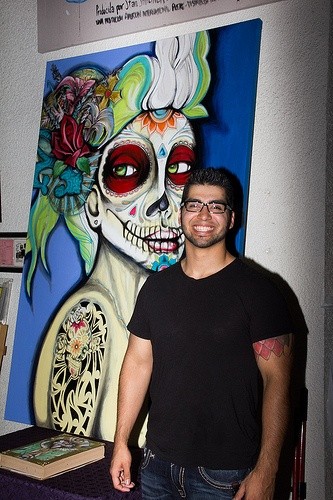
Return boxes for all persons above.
[109,167,308,500]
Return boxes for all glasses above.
[181,199,232,214]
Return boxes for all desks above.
[0,419,143,500]
